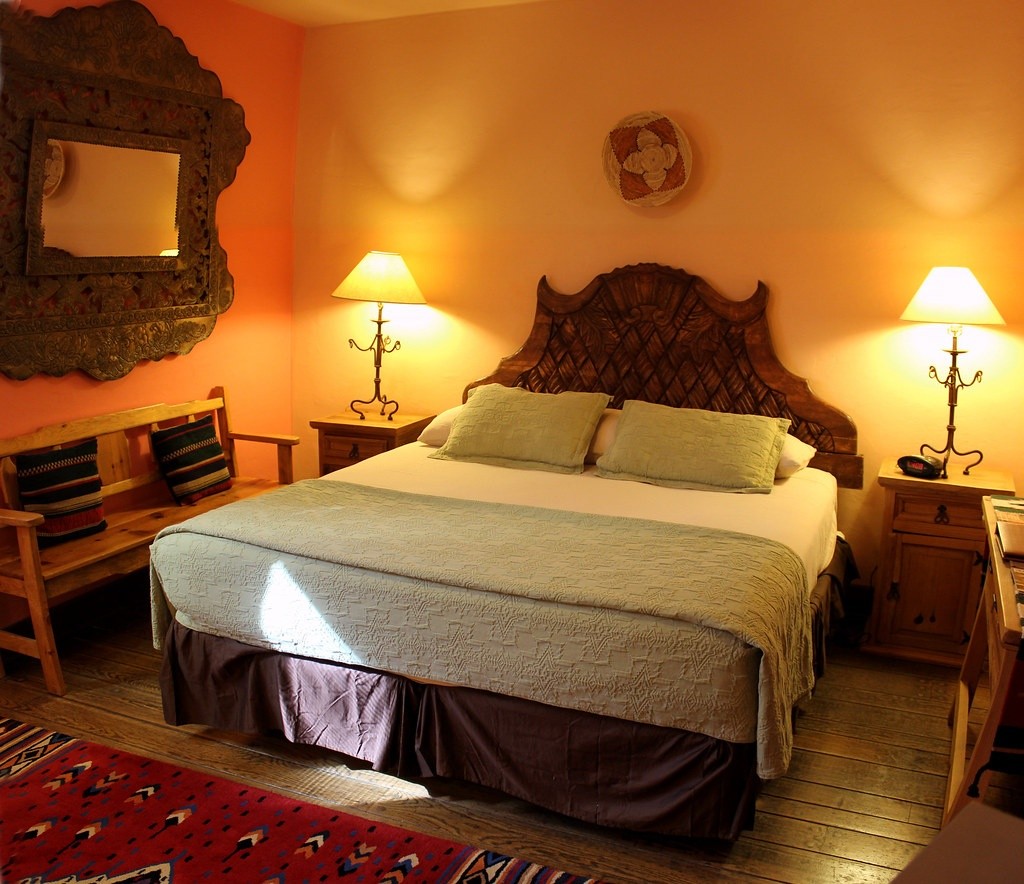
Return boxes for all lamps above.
[900,266,1006,478]
[330,250,428,422]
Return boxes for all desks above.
[939,494,1024,827]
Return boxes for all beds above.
[142,261,866,868]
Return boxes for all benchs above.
[0,386,302,698]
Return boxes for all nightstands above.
[859,457,1017,668]
[309,410,437,479]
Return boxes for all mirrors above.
[0,0,253,384]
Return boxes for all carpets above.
[0,715,603,884]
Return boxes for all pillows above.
[417,403,818,482]
[8,439,109,551]
[427,383,615,477]
[150,414,233,509]
[592,400,793,494]
